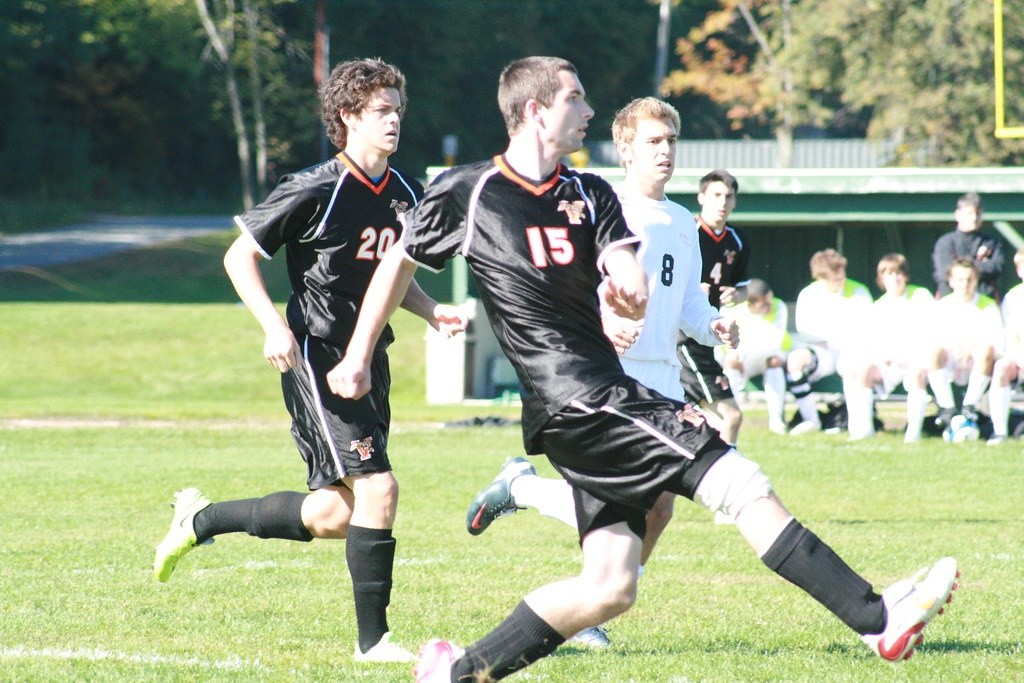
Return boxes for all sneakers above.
[935,407,959,428]
[862,556,961,663]
[153,488,216,584]
[465,454,537,535]
[961,405,979,422]
[567,627,612,646]
[354,631,417,661]
[414,637,466,683]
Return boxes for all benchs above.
[746,371,1024,402]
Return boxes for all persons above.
[716,248,1024,446]
[327,57,962,683]
[154,58,469,664]
[676,170,745,524]
[932,193,1007,305]
[466,96,739,652]
